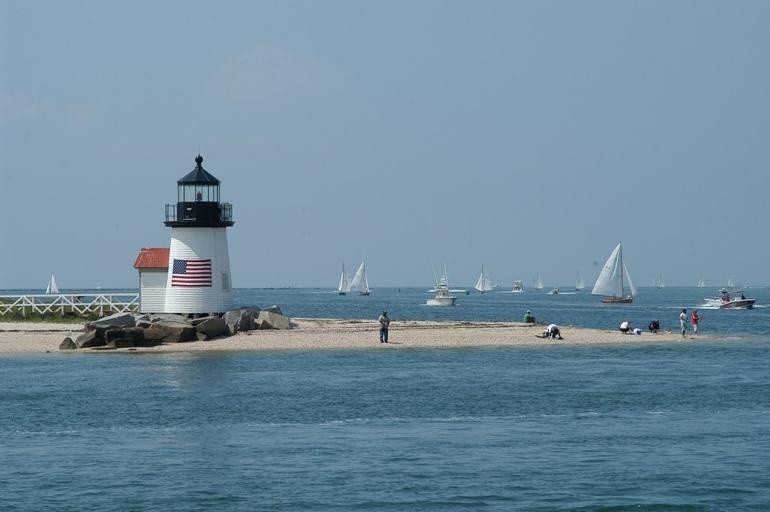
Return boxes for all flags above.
[171,258,213,289]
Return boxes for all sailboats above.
[46,273,60,294]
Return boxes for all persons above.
[523,308,536,323]
[378,309,390,343]
[544,324,564,340]
[619,320,630,334]
[690,309,700,338]
[679,308,689,339]
[648,319,660,334]
